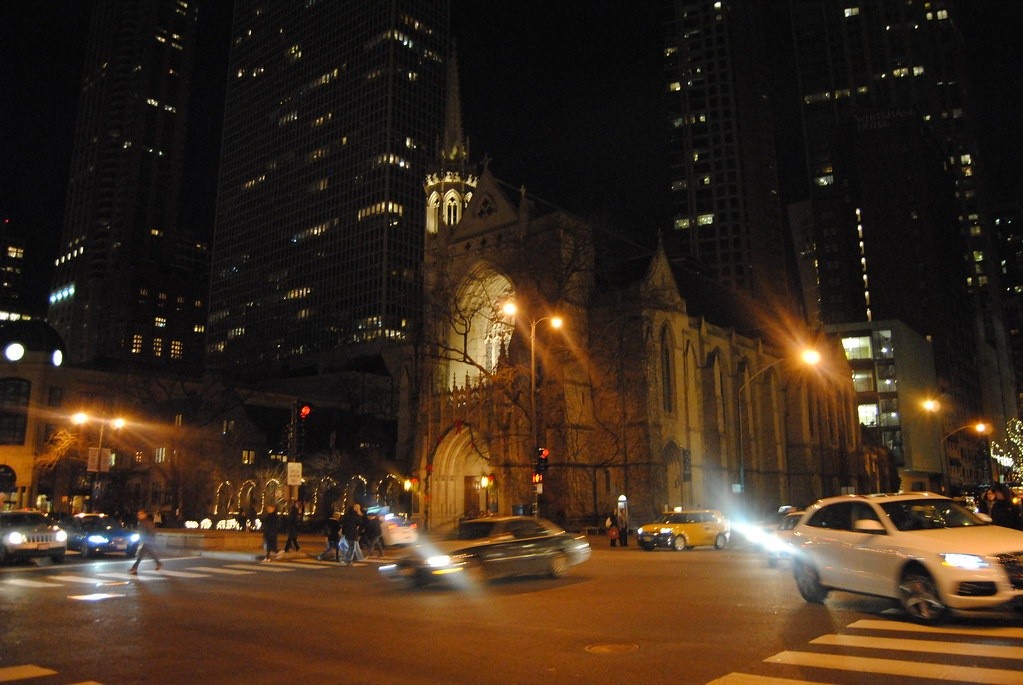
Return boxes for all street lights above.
[877,398,933,492]
[503,300,562,519]
[940,422,984,495]
[737,347,820,507]
[72,411,125,514]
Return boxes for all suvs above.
[790,490,1023,625]
[636,509,730,551]
[0,511,67,567]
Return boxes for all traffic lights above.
[532,472,543,483]
[297,402,311,437]
[536,448,548,473]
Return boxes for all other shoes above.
[261,558,270,562]
[155,562,162,570]
[274,550,283,557]
[127,566,136,574]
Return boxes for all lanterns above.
[487,475,494,481]
[302,406,310,414]
[533,472,543,483]
[424,493,431,501]
[410,476,418,484]
[543,448,549,456]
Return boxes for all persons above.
[618,508,628,546]
[991,484,1023,531]
[284,506,301,553]
[356,509,371,549]
[980,489,999,515]
[128,508,164,573]
[342,503,366,564]
[361,513,385,558]
[317,512,342,562]
[607,524,619,547]
[610,508,618,526]
[260,504,282,562]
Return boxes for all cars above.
[394,516,591,590]
[749,511,806,568]
[53,513,140,559]
[338,515,417,549]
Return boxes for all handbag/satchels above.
[337,536,348,553]
[609,528,619,540]
